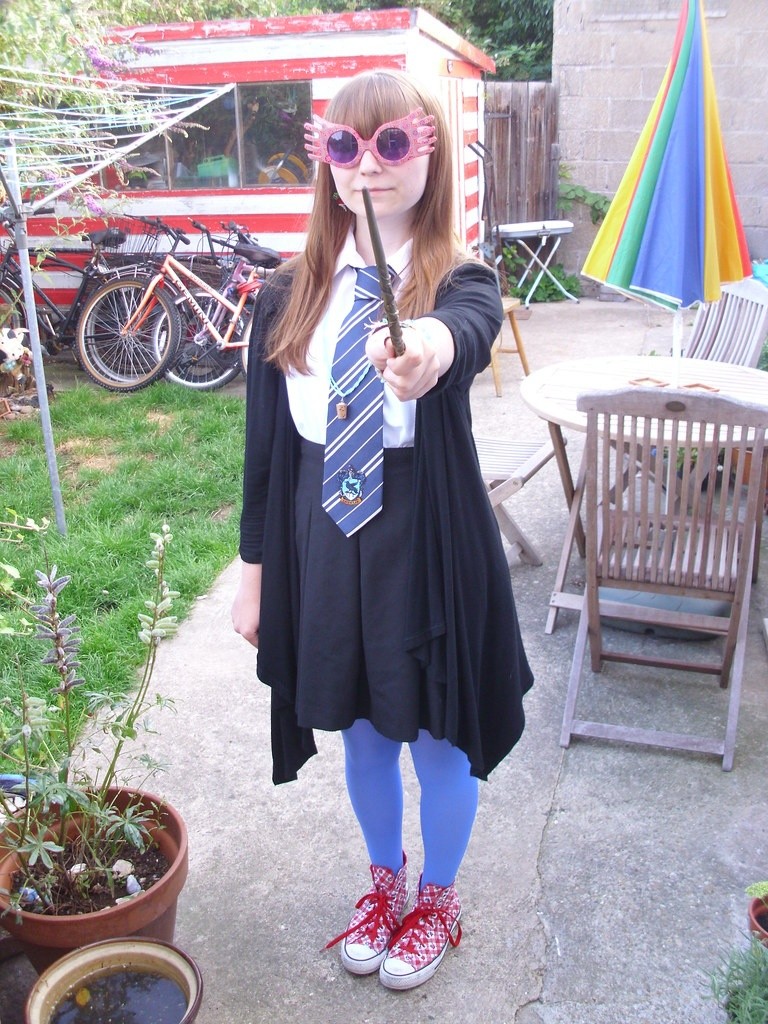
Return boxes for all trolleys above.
[466,139,533,322]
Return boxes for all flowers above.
[0,507,189,926]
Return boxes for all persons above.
[232,74,534,992]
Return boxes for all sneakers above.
[380,873,462,990]
[319,850,410,974]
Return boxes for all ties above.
[322,265,399,538]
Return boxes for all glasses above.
[303,105,437,170]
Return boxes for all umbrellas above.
[580,0,755,385]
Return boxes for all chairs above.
[487,297,531,397]
[597,278,768,519]
[559,386,768,773]
[473,434,567,566]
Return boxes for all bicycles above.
[0,206,289,393]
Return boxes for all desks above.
[491,221,580,309]
[520,355,768,634]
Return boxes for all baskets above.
[105,211,161,263]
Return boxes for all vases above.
[0,786,190,977]
[25,937,203,1024]
[731,448,768,488]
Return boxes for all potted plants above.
[663,446,726,491]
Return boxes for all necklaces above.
[329,363,371,420]
